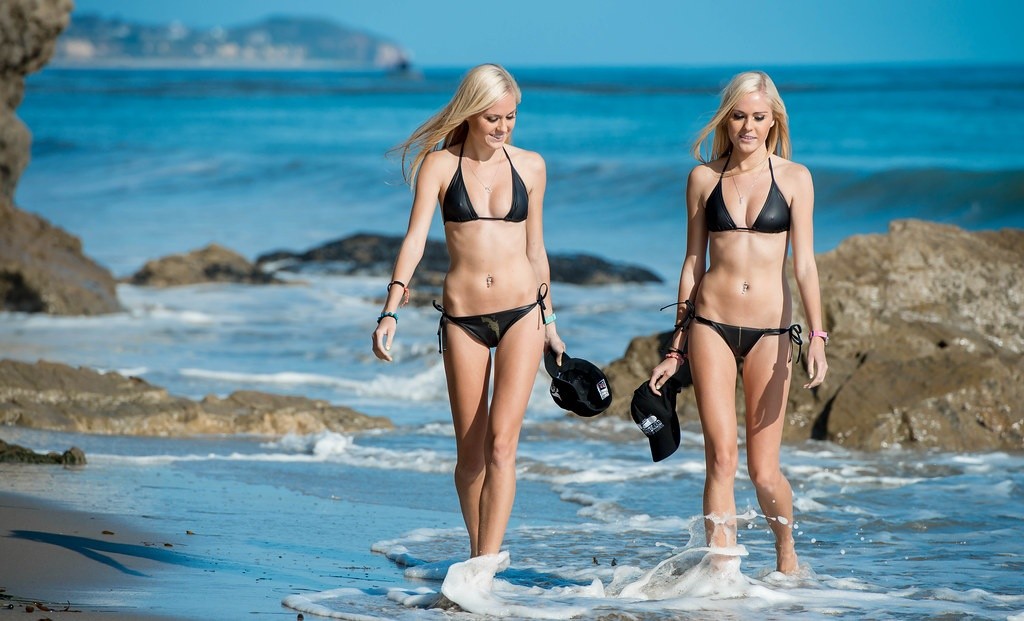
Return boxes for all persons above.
[372,64,566,559]
[649,71,828,587]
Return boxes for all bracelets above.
[808,330,828,346]
[665,345,686,365]
[544,313,556,324]
[387,280,409,308]
[377,312,398,323]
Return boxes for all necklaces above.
[461,147,504,193]
[730,158,768,203]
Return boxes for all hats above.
[631,375,683,462]
[544,349,612,417]
